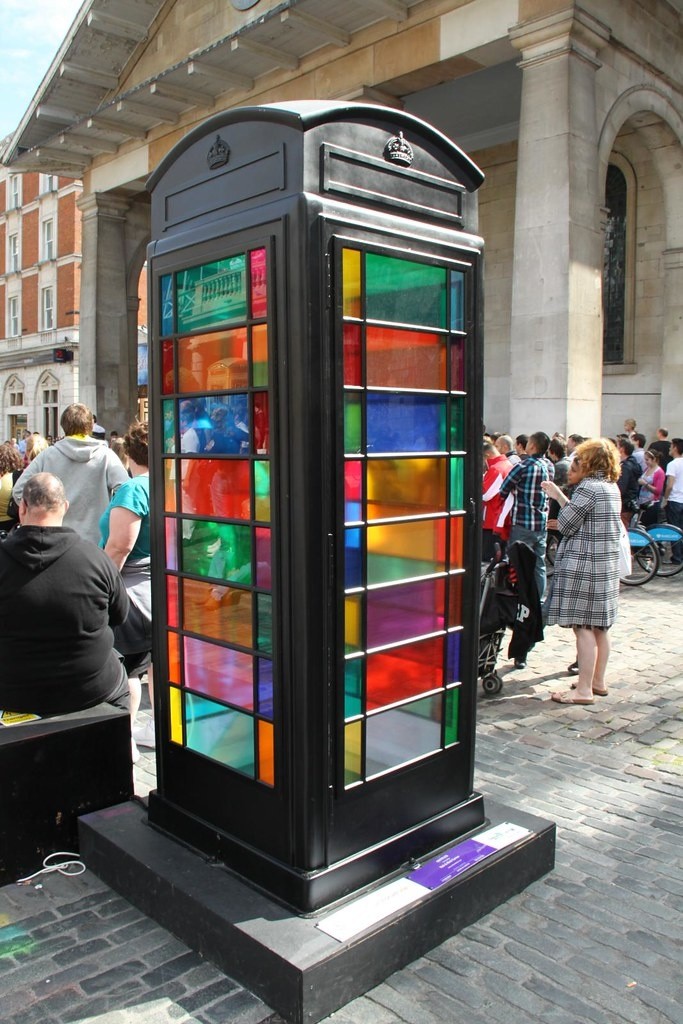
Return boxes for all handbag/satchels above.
[7,470,24,519]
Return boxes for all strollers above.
[481,541,522,693]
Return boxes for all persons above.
[164,397,275,611]
[482,417,683,705]
[0,403,153,764]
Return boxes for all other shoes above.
[567,663,579,676]
[514,658,527,669]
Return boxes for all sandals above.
[551,691,596,705]
[570,682,609,697]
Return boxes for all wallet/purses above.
[637,498,659,510]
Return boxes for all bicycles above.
[546,527,661,585]
[625,499,683,577]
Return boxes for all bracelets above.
[645,483,649,487]
